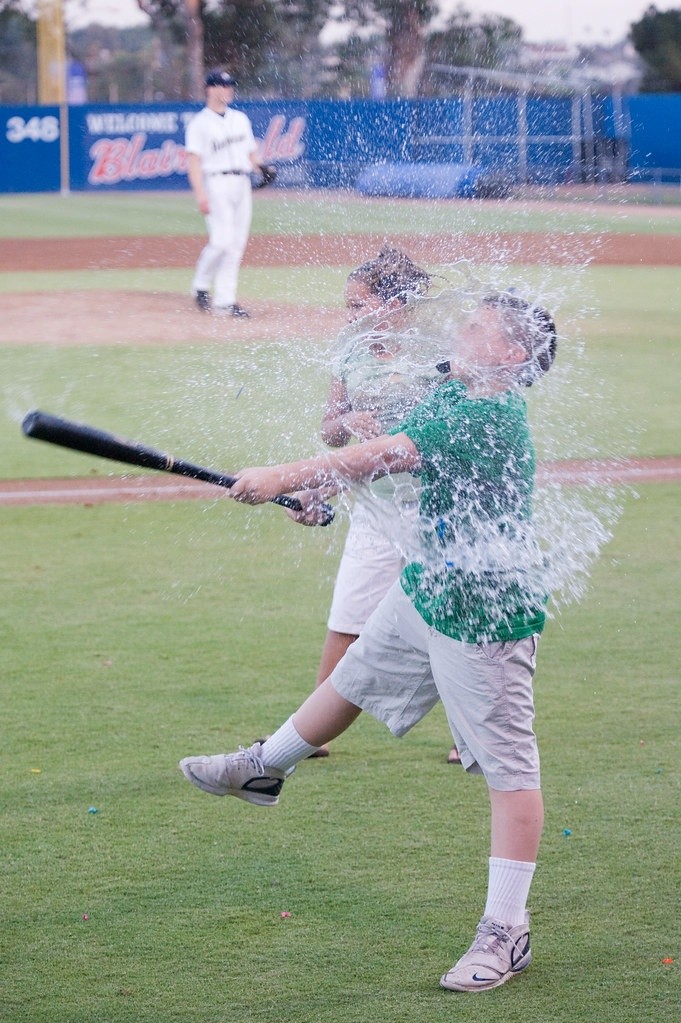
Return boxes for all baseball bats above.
[20,409,335,529]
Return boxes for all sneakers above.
[440,910,533,992]
[179,742,296,806]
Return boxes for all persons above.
[181,293,558,995]
[183,72,278,318]
[245,249,468,765]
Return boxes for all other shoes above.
[254,737,330,757]
[216,304,251,319]
[195,290,212,315]
[448,745,459,765]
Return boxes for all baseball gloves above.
[253,163,278,190]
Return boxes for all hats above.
[205,70,236,86]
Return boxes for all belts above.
[221,169,246,175]
